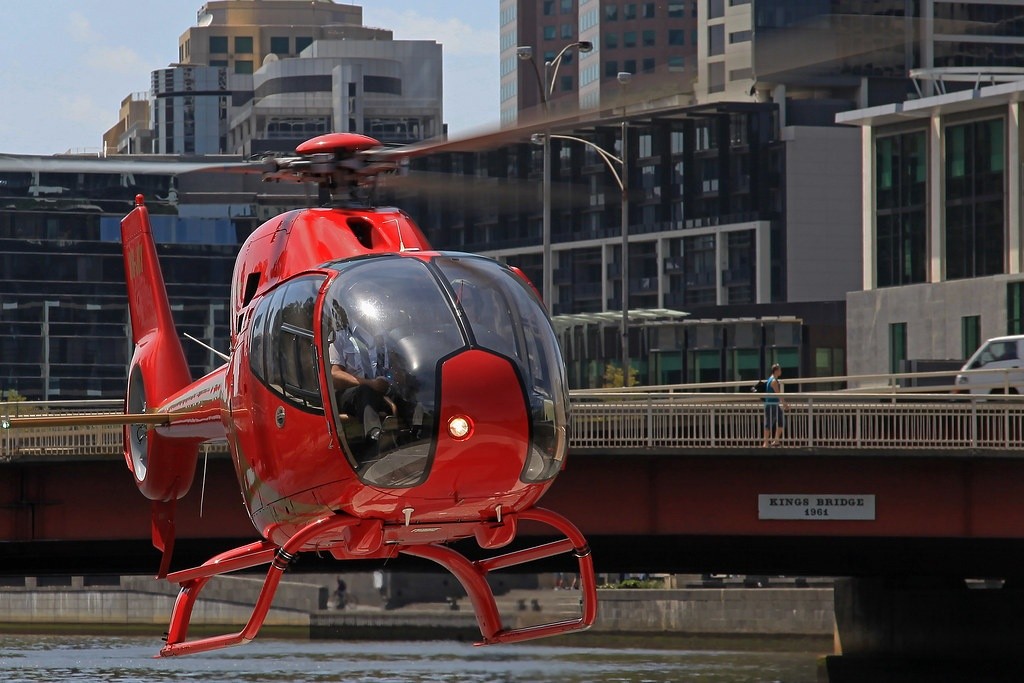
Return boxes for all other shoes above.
[367,429,381,440]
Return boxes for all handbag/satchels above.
[754,382,769,402]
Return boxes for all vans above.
[950,333,1024,405]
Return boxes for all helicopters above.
[0,1,1001,660]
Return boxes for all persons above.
[988,342,1017,361]
[763,364,789,448]
[327,298,423,450]
[421,280,533,439]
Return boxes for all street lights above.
[529,71,634,387]
[514,39,594,324]
